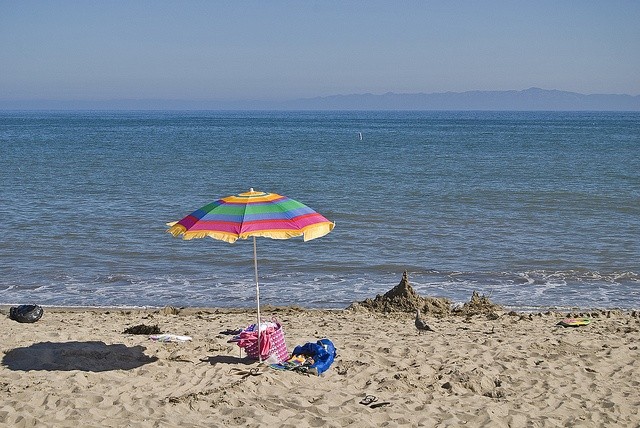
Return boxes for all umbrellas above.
[165,187,336,364]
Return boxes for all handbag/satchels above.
[228,317,292,366]
